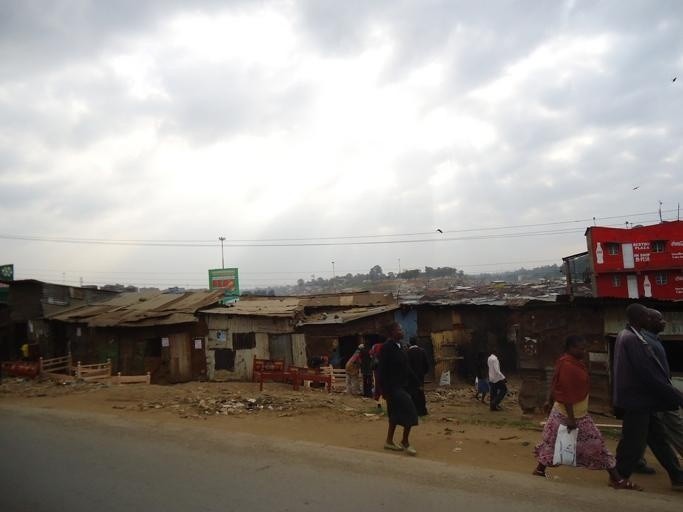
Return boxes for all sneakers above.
[607,459,656,490]
[531,468,546,477]
[383,441,417,456]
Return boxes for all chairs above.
[319,366,350,390]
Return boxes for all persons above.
[327,323,433,454]
[532,335,643,492]
[475,357,489,406]
[634,309,682,474]
[487,346,507,410]
[610,303,683,490]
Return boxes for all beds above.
[250,354,300,392]
[40,349,110,382]
[74,354,153,384]
[285,359,330,393]
